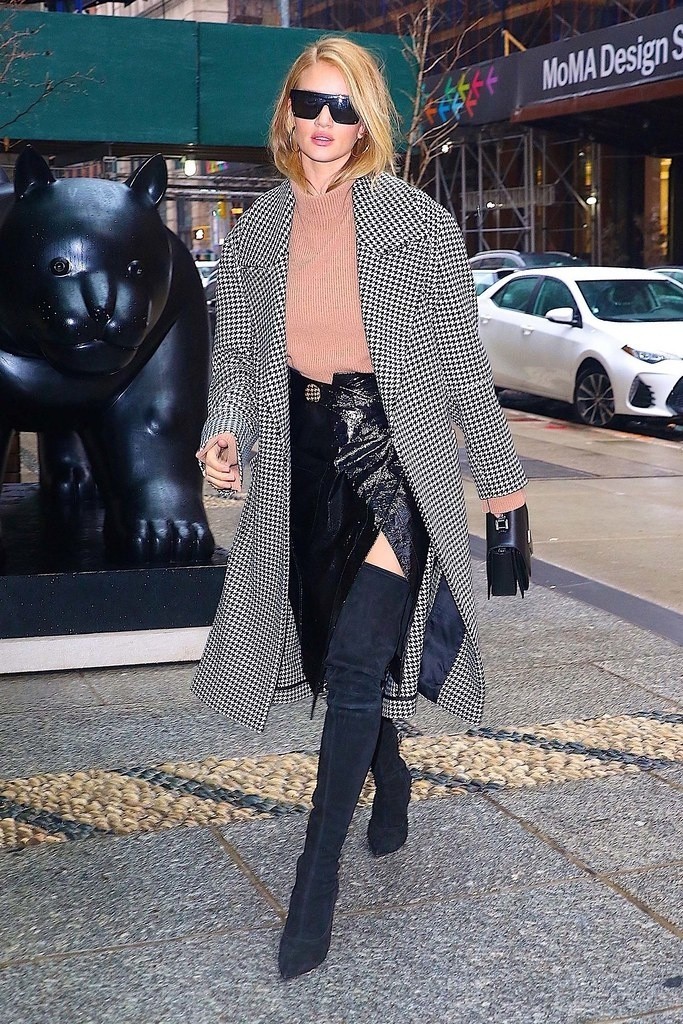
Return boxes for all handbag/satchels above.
[486,503,533,600]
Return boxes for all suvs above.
[469,249,598,310]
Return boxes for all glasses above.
[288,89,363,125]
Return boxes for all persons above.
[196,34,532,985]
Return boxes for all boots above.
[277,561,419,980]
[369,716,411,857]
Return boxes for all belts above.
[289,373,364,416]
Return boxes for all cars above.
[477,266,683,435]
